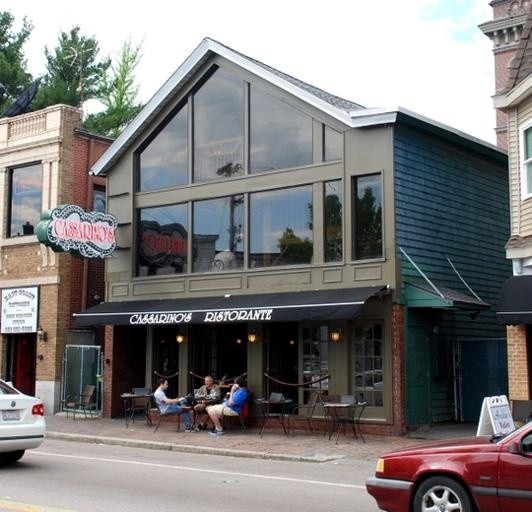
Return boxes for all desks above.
[255,399,292,436]
[186,399,218,433]
[324,402,369,444]
[121,393,156,425]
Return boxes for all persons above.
[205,376,248,436]
[194,376,221,432]
[153,378,200,432]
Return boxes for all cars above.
[1,377,47,467]
[312,369,384,393]
[366,416,532,511]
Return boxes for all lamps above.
[37,325,47,343]
[176,334,184,344]
[329,327,343,344]
[247,329,257,343]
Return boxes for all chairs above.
[318,395,348,437]
[208,259,224,271]
[125,396,150,428]
[287,393,319,437]
[221,398,247,433]
[153,398,180,433]
[67,385,95,419]
